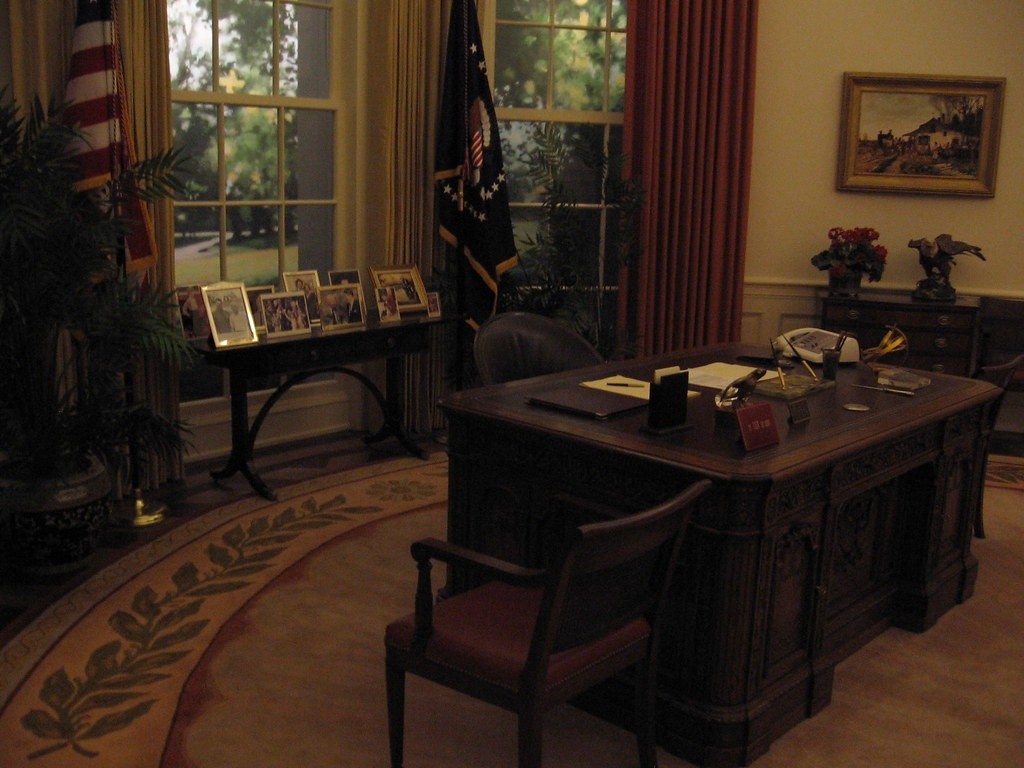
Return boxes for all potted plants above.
[0,82,204,577]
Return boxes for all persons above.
[381,289,396,317]
[182,279,362,337]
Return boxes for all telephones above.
[776,328,859,364]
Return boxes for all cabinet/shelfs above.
[814,290,982,377]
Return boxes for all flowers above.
[810,227,887,283]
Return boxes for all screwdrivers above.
[852,384,914,396]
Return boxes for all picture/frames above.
[367,263,428,314]
[316,283,365,331]
[199,282,259,348]
[835,72,1007,198]
[282,270,322,323]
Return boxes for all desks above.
[437,340,1005,768]
[183,310,461,502]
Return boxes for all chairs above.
[384,480,713,768]
[969,353,1024,539]
[469,311,605,388]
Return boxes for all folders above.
[525,386,649,420]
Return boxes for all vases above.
[828,269,863,296]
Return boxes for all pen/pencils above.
[835,330,849,351]
[781,333,816,378]
[770,338,786,390]
[607,382,645,388]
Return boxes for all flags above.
[60,0,160,288]
[436,0,516,334]
